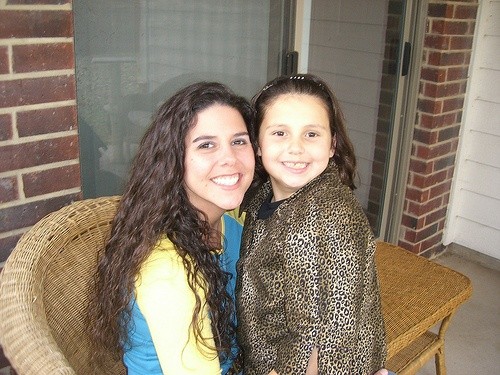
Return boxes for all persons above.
[237,73,385,375]
[80,82,388,375]
